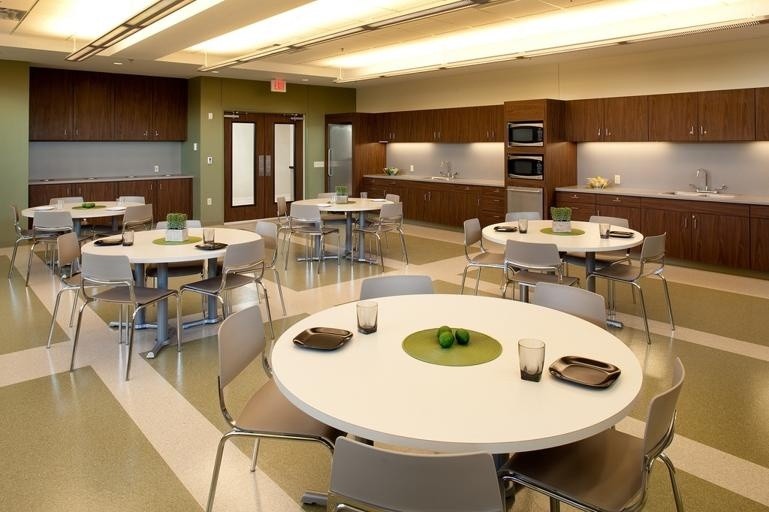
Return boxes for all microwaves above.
[508,154,544,180]
[509,121,543,147]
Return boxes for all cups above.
[361,192,367,200]
[202,229,214,243]
[56,200,63,210]
[115,198,124,207]
[329,195,335,204]
[354,301,378,335]
[121,230,133,246]
[518,338,545,382]
[599,224,610,240]
[517,218,528,234]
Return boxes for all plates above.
[292,327,353,351]
[371,199,386,202]
[314,203,330,207]
[193,243,227,250]
[548,355,620,389]
[93,238,121,246]
[29,205,55,211]
[608,230,634,238]
[493,225,516,232]
[105,207,126,211]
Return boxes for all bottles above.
[439,160,448,177]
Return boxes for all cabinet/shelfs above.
[361,178,505,231]
[455,105,504,143]
[28,177,192,218]
[560,96,649,141]
[756,88,769,141]
[649,90,756,144]
[688,200,751,272]
[751,205,769,275]
[365,113,410,142]
[411,108,455,143]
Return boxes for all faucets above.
[696,168,708,186]
[440,160,451,177]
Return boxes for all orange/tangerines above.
[82,203,95,208]
[437,326,470,348]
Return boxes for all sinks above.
[698,193,737,198]
[657,190,701,196]
[424,177,464,182]
